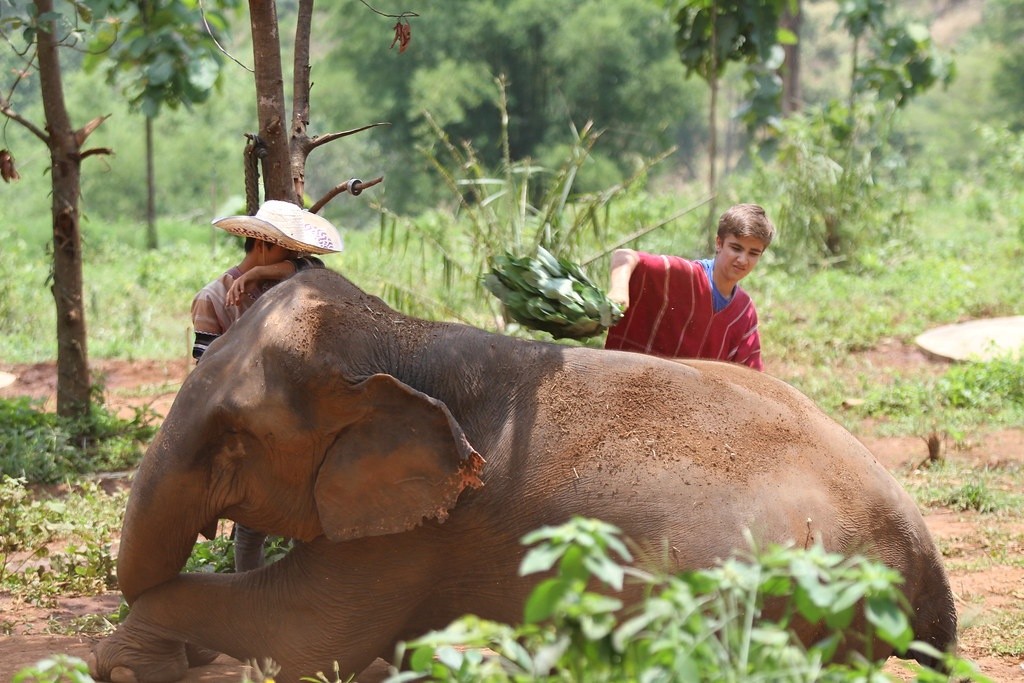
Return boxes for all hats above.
[211,200,343,255]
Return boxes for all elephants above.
[89,268,958,682]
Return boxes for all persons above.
[605,203,773,373]
[191,199,343,574]
